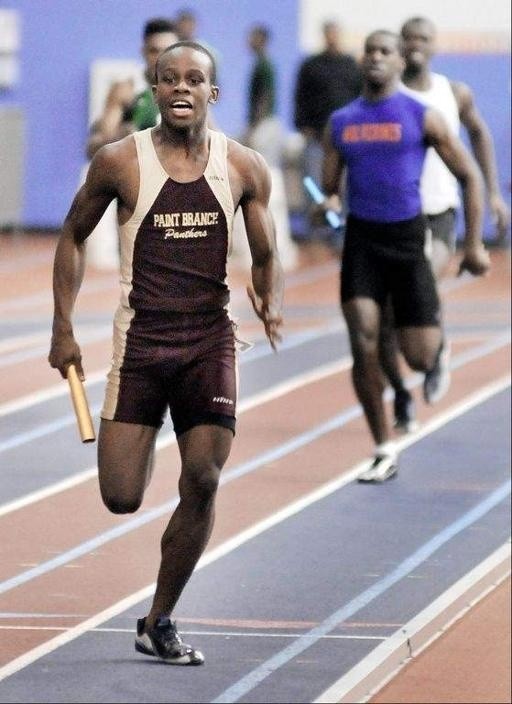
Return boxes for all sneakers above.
[391,391,418,434]
[355,442,399,482]
[423,335,452,405]
[133,614,206,666]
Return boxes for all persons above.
[48,39,284,665]
[176,7,362,273]
[311,30,491,482]
[394,19,510,277]
[86,17,220,160]
